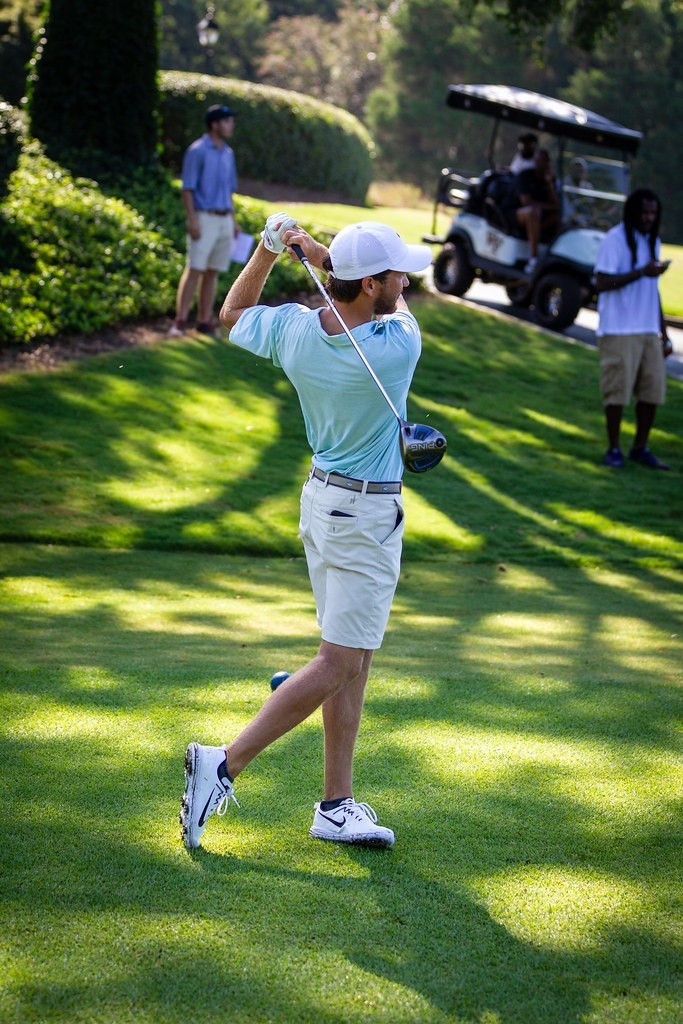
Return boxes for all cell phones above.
[662,260,672,266]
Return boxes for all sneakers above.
[178,742,240,848]
[309,798,394,848]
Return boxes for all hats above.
[327,221,433,281]
[518,133,537,145]
[205,104,241,126]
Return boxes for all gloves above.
[261,211,297,255]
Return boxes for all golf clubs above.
[276,214,455,481]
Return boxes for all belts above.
[206,209,231,216]
[310,465,401,494]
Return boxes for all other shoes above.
[525,259,539,275]
[628,445,669,470]
[196,323,220,338]
[604,448,624,469]
[168,326,185,339]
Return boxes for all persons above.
[594,189,674,470]
[168,103,241,338]
[177,212,432,851]
[472,132,560,273]
